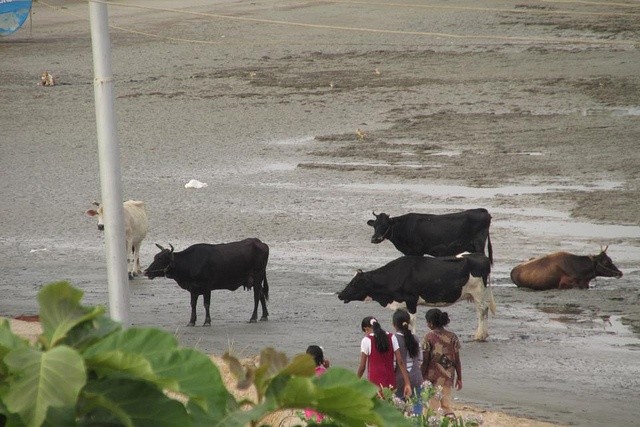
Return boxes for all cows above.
[143,238,269,327]
[367,208,494,264]
[340,251,496,341]
[510,244,623,289]
[87,200,148,280]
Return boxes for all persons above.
[421,309,464,419]
[393,309,425,416]
[304,345,330,424]
[356,316,412,400]
